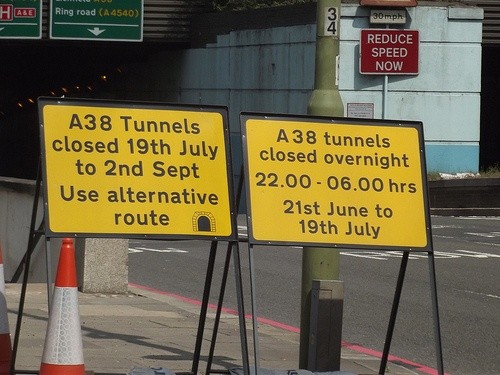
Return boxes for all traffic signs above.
[0,0,143,43]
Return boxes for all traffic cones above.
[39,237,86,375]
[0,244,16,374]
